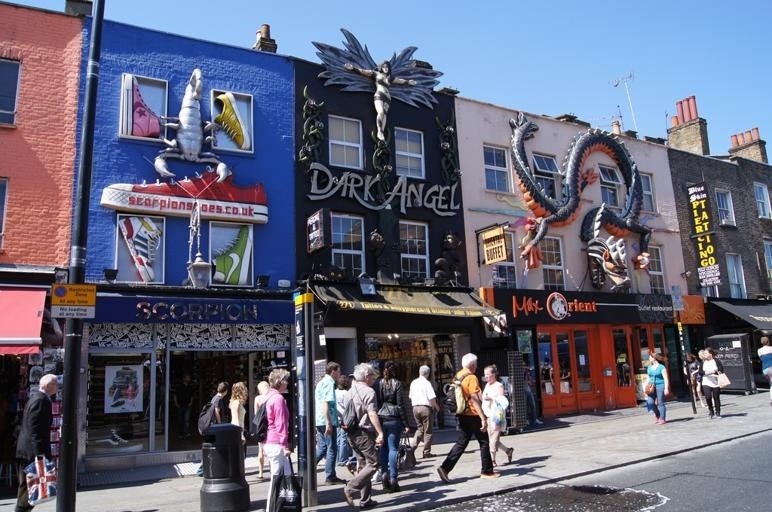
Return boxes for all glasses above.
[50,381,59,386]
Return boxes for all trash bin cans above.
[200,424,250,512]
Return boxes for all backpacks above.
[249,392,276,443]
[340,386,365,434]
[443,372,473,416]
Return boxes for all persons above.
[14,373,59,512]
[698,348,724,420]
[683,352,705,409]
[229,382,249,448]
[408,364,440,459]
[698,350,704,362]
[171,374,193,440]
[481,365,514,467]
[343,362,385,510]
[344,60,417,142]
[618,362,626,387]
[254,380,270,484]
[335,374,351,467]
[542,358,553,385]
[524,364,543,426]
[757,336,772,406]
[436,353,501,483]
[373,361,407,492]
[196,381,229,477]
[647,352,670,425]
[315,361,343,486]
[260,368,294,512]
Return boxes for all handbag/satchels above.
[22,455,58,506]
[715,368,731,388]
[690,376,696,386]
[397,431,417,471]
[560,380,570,394]
[524,373,532,393]
[196,402,217,437]
[269,455,304,511]
[646,373,656,395]
[545,382,554,395]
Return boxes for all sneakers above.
[256,474,264,482]
[113,387,121,402]
[508,448,514,463]
[480,471,502,478]
[717,416,722,420]
[118,217,162,282]
[111,399,125,407]
[437,466,452,484]
[654,418,665,426]
[211,226,252,286]
[122,73,161,137]
[707,414,715,420]
[343,488,354,507]
[85,428,145,454]
[100,165,269,224]
[214,91,251,150]
[492,461,497,467]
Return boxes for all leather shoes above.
[423,454,437,458]
[358,500,378,511]
[325,478,347,485]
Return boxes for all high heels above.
[120,400,137,412]
[380,475,389,489]
[390,478,400,493]
